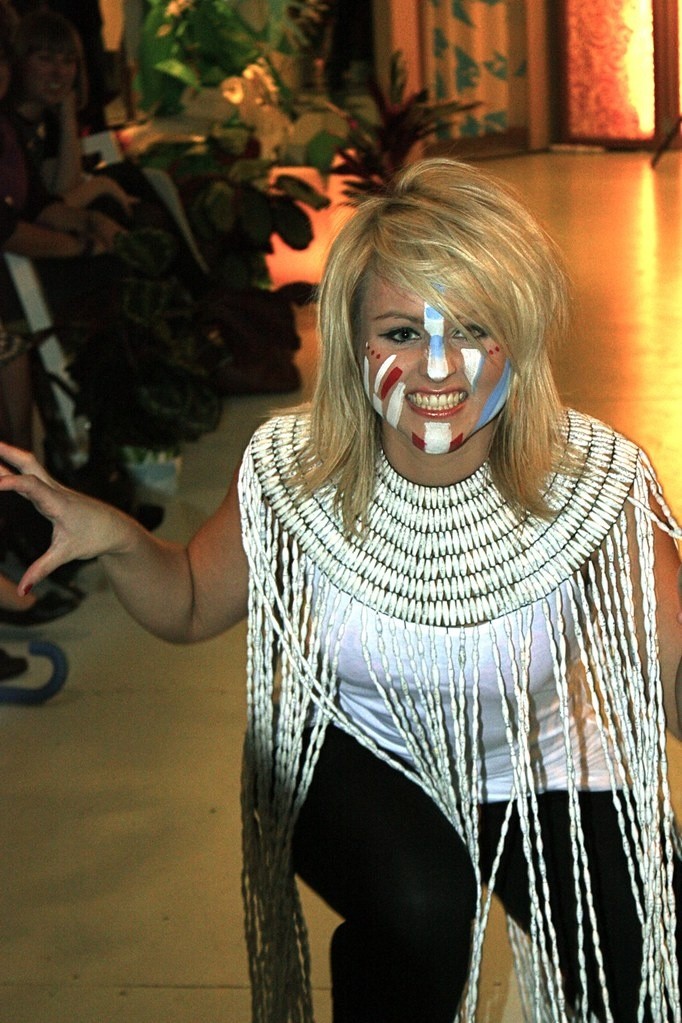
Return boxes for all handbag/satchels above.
[190,288,303,396]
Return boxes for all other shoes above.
[1,590,77,626]
[0,650,27,679]
[134,504,164,531]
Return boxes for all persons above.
[0,157,682,1023]
[0,0,167,627]
